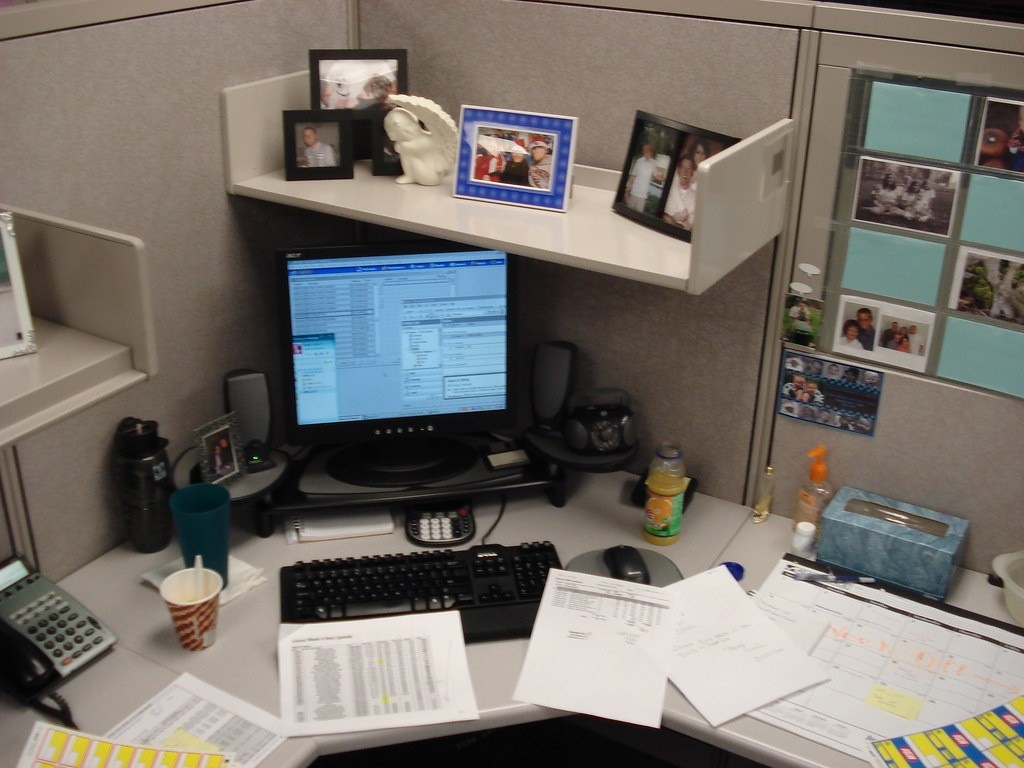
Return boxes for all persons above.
[319,62,395,112]
[663,138,716,232]
[879,321,924,354]
[784,297,819,349]
[298,128,336,167]
[623,142,683,212]
[475,136,553,188]
[780,356,881,432]
[861,173,939,225]
[839,307,874,352]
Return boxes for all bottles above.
[645,441,683,545]
[112,416,174,553]
[794,522,817,558]
[753,466,775,525]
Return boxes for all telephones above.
[0,556,117,698]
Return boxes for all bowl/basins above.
[991,550,1024,629]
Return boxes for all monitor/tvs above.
[272,241,517,488]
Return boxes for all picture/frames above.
[307,47,412,163]
[448,101,579,214]
[0,210,39,360]
[279,108,354,182]
[611,106,746,246]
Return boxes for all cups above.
[170,483,232,590]
[160,567,223,648]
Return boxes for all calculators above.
[406,494,476,546]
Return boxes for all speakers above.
[531,340,580,441]
[224,368,276,474]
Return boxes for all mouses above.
[603,544,652,587]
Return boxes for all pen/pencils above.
[795,571,875,583]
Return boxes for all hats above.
[529,134,549,150]
[514,138,526,148]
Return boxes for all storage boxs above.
[815,485,972,605]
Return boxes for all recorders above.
[563,386,638,456]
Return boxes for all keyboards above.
[280,540,565,643]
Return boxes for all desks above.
[0,648,320,768]
[662,498,1024,768]
[60,474,761,756]
